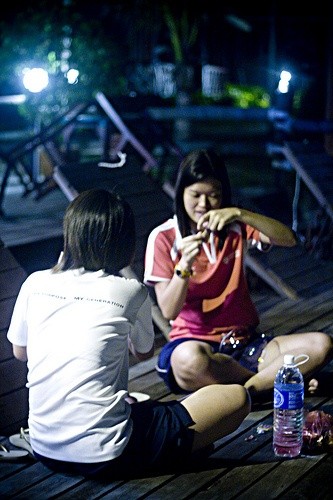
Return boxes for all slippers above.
[9,427,34,456]
[0,445,29,458]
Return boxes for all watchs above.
[175,264,195,279]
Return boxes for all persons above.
[7,189,251,481]
[144,148,332,401]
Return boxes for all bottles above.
[273,355,310,457]
